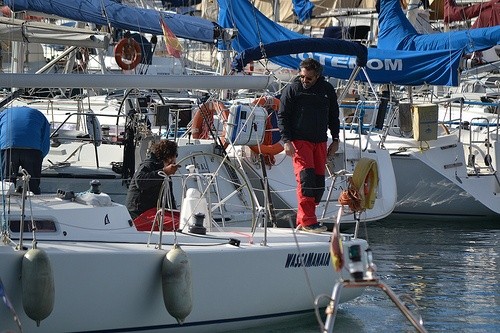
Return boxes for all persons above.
[125,139,183,232]
[276,58,340,233]
[0,106,51,195]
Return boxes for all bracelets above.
[332,137,340,142]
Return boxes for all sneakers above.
[302,224,327,232]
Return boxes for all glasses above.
[300,73,316,81]
[175,153,178,158]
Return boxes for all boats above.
[0,0,499,333]
